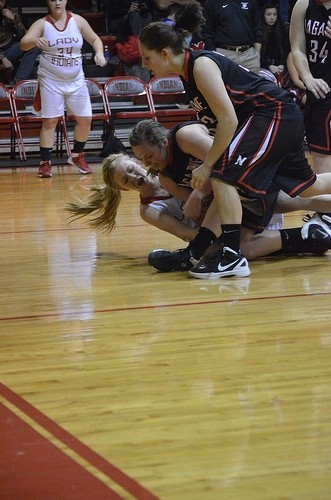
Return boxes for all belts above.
[215,44,252,53]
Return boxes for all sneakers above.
[300,212,331,255]
[148,245,199,272]
[188,242,251,280]
[66,152,92,174]
[38,160,52,178]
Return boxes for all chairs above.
[0,12,279,162]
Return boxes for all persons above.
[0,0,39,116]
[20,0,107,178]
[64,0,331,279]
